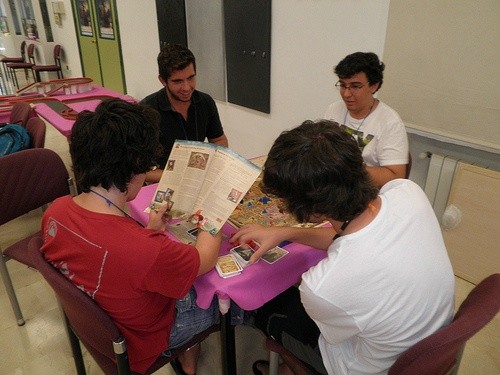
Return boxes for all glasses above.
[334,80,369,90]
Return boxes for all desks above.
[34,86,137,136]
[128,155,330,375]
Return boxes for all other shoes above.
[253,360,270,375]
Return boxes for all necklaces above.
[89,189,145,227]
[344,99,375,138]
[333,203,375,240]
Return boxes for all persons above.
[40,99,239,375]
[136,45,228,184]
[323,52,409,188]
[229,118,455,375]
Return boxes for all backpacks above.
[0,120,31,156]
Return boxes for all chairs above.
[263,273,500,375]
[0,102,76,326]
[27,237,222,375]
[6,44,35,90]
[2,41,29,80]
[31,44,64,82]
